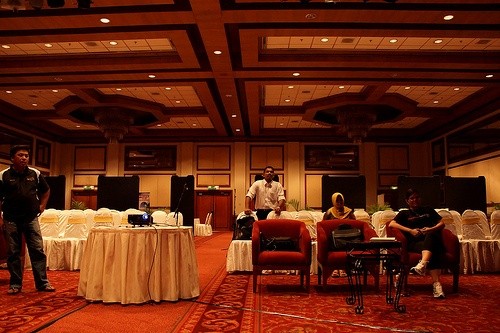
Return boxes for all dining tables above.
[77,226,199,305]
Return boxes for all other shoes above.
[37,285,56,292]
[7,287,22,294]
[332,269,348,277]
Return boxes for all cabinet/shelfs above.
[193,190,234,231]
[69,189,97,210]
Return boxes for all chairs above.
[25,209,212,271]
[226,209,500,293]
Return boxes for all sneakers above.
[411,260,429,277]
[432,282,445,298]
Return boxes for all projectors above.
[128,214,153,227]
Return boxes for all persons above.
[0,145,55,294]
[245,166,285,220]
[388,188,446,299]
[322,192,355,277]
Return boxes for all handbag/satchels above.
[267,239,298,251]
[332,224,362,250]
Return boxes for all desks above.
[341,239,406,313]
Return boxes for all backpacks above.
[237,216,255,240]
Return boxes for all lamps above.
[93,109,135,144]
[336,111,377,143]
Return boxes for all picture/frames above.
[305,144,359,171]
[123,145,179,174]
[0,126,32,164]
